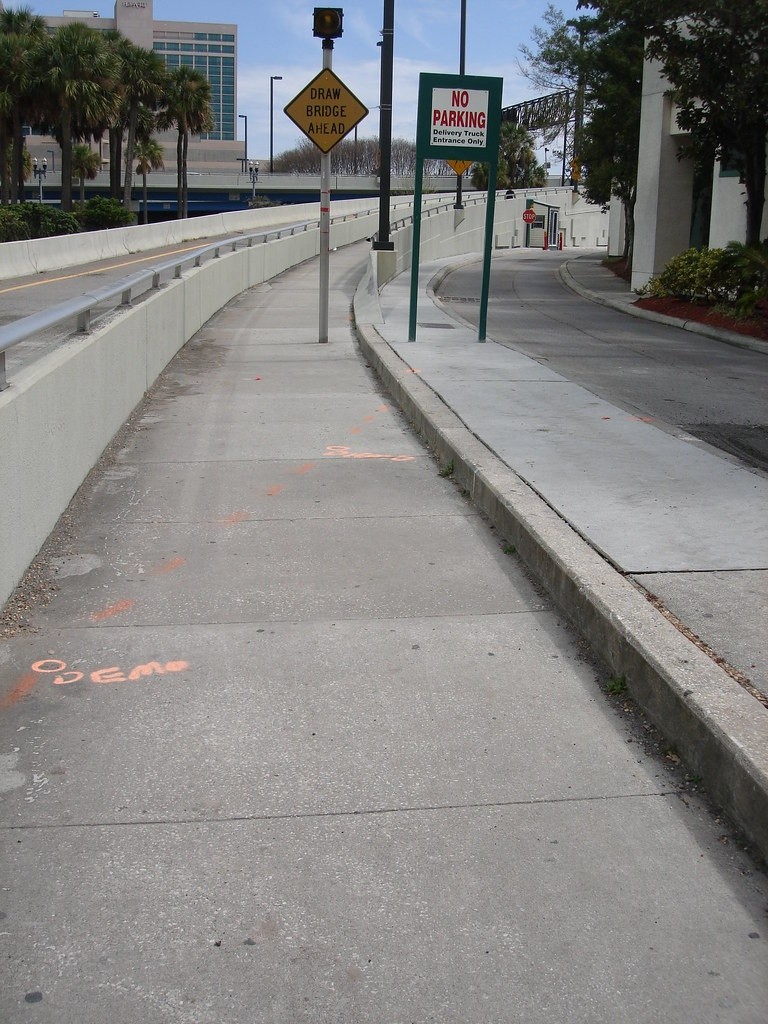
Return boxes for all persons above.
[506,186,516,199]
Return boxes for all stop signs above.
[523,209,537,224]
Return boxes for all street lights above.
[237,158,249,172]
[239,115,247,172]
[270,76,282,172]
[246,159,263,199]
[32,156,47,203]
[545,148,549,180]
[47,149,54,171]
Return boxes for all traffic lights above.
[312,7,344,38]
